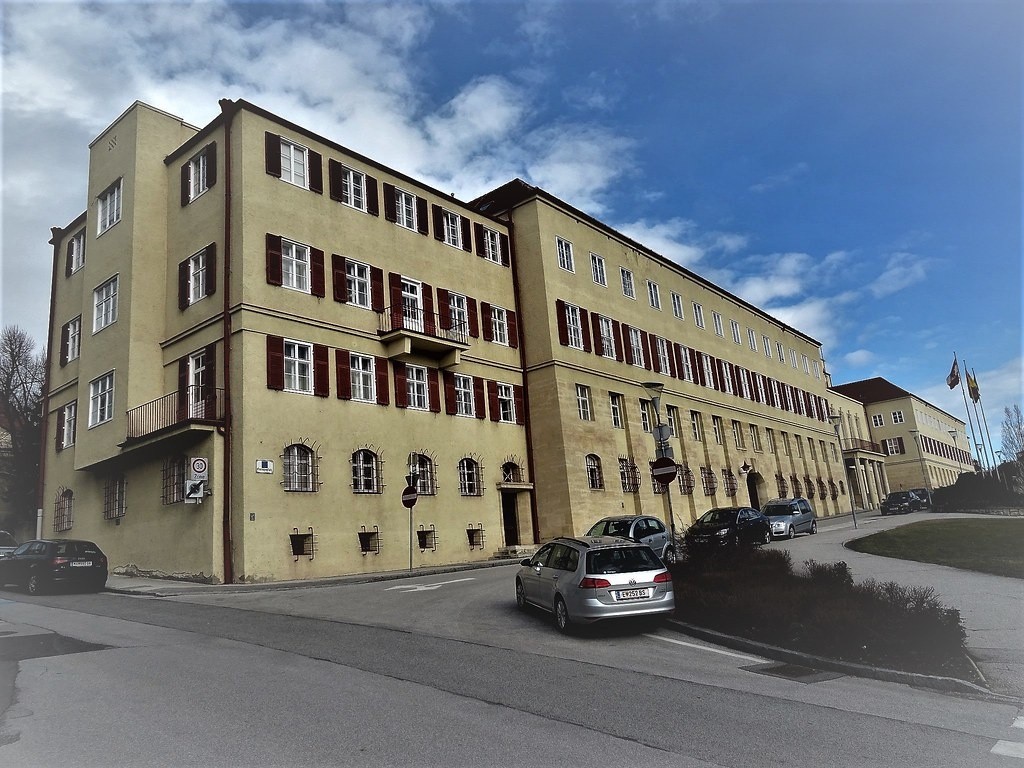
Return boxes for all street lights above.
[828,414,859,528]
[995,449,1004,465]
[976,444,986,472]
[640,382,680,564]
[908,429,931,493]
[949,429,963,474]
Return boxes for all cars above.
[0,539,109,594]
[0,530,19,558]
[517,533,678,635]
[684,506,772,553]
[911,487,932,507]
[879,490,923,517]
[582,515,677,564]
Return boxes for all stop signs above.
[401,486,419,509]
[651,456,678,485]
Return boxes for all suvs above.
[761,498,819,540]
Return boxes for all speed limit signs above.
[191,457,209,481]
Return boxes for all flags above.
[970,373,981,405]
[945,358,960,390]
[965,370,977,397]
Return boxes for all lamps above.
[739,461,750,475]
